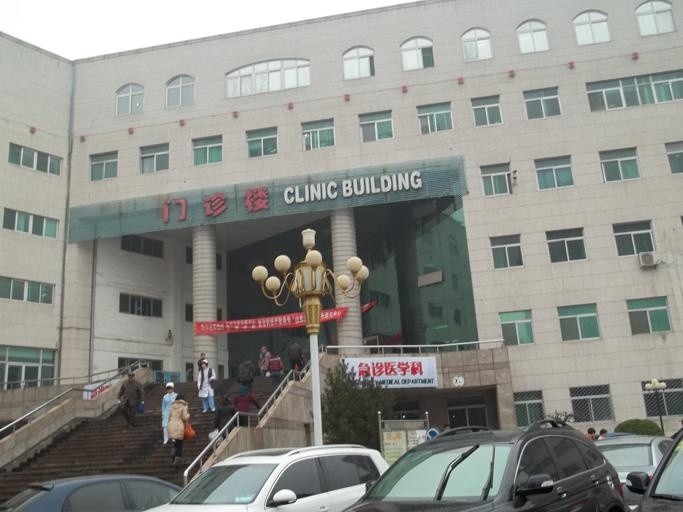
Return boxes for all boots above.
[173,456,182,470]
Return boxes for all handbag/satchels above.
[138,401,145,413]
[186,422,196,438]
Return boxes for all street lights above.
[642,375,668,435]
[250,226,374,444]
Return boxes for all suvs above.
[339,416,626,511]
[139,440,391,511]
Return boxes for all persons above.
[597,428,607,439]
[117,372,145,430]
[443,425,451,430]
[585,427,596,439]
[160,340,304,468]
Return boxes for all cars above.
[0,471,183,511]
[591,428,682,512]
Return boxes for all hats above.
[166,382,174,389]
